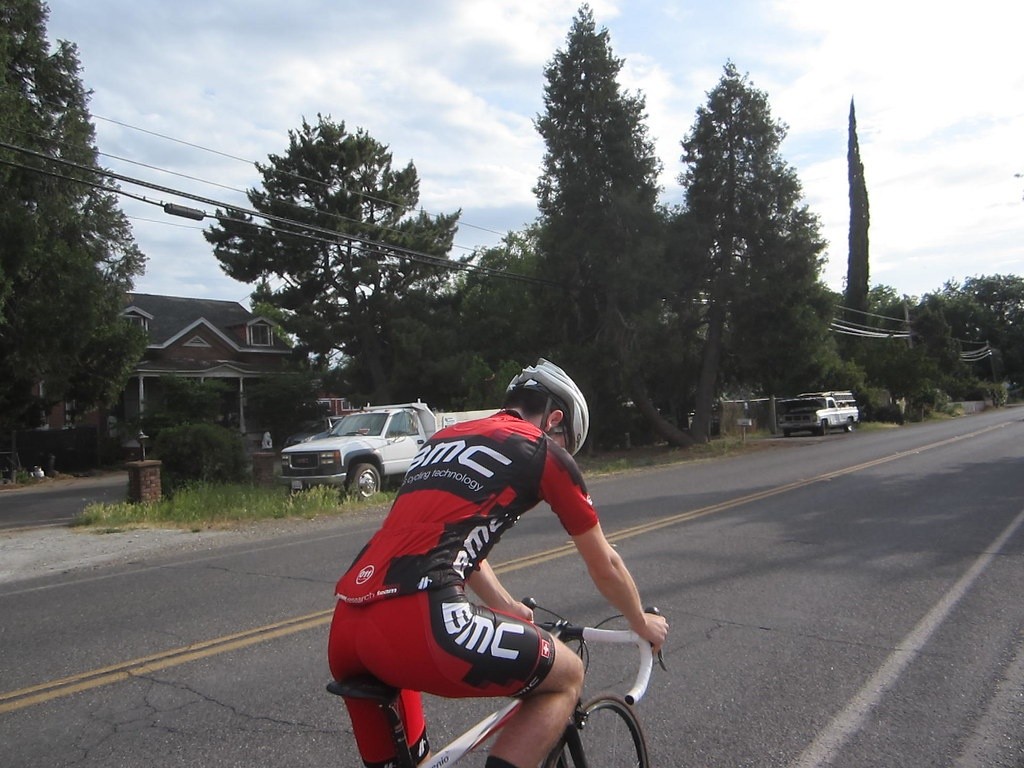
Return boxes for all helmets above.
[505,358,590,457]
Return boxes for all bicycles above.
[326,593,669,768]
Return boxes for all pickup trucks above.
[777,390,861,437]
[274,397,507,503]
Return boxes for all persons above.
[328,354,670,768]
